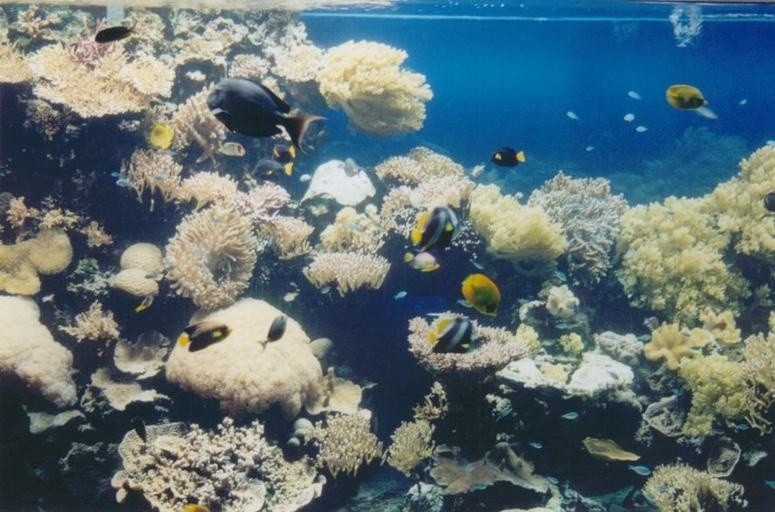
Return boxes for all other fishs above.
[94,21,771,511]
